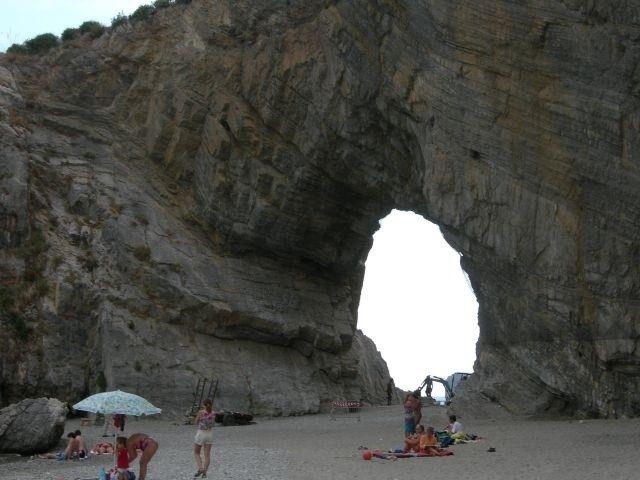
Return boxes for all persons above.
[412,389,422,426]
[401,413,465,457]
[37,431,80,463]
[423,375,433,397]
[116,433,159,480]
[193,398,217,478]
[72,430,88,461]
[404,391,422,438]
[93,441,115,455]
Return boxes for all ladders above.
[188,378,219,424]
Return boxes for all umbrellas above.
[71,389,162,473]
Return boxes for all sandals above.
[202,473,206,478]
[194,469,204,477]
